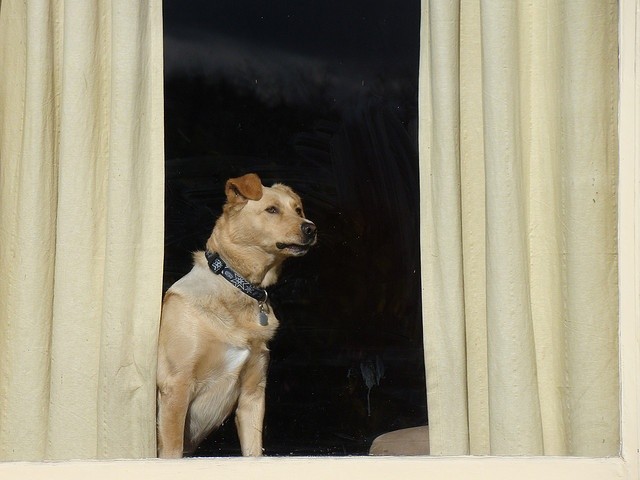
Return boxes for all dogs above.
[157,172,317,458]
